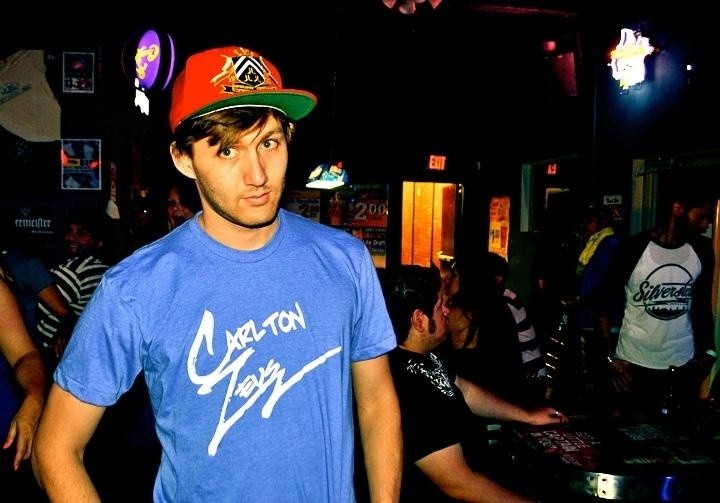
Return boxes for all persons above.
[0,170,207,366]
[382,263,570,503]
[30,43,404,503]
[595,167,716,397]
[0,274,48,472]
[370,170,627,421]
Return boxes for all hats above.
[169,45,318,134]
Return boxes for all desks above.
[499,402,720,503]
